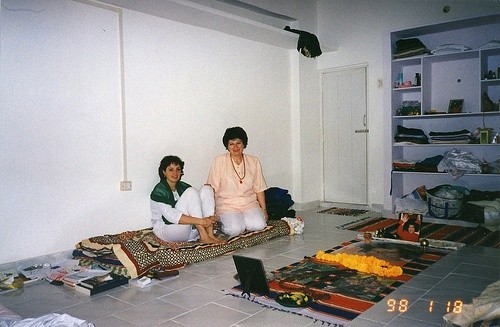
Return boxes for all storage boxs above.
[282,217,304,235]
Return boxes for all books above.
[0,266,129,297]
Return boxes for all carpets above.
[224,207,491,327]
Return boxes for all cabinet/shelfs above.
[384,12,500,212]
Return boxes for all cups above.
[364,232,372,243]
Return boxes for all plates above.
[275,292,313,308]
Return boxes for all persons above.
[150,156,226,244]
[207,126,267,236]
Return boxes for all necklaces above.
[230,153,245,184]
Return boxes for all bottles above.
[414,73,420,86]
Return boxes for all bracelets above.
[261,208,267,211]
